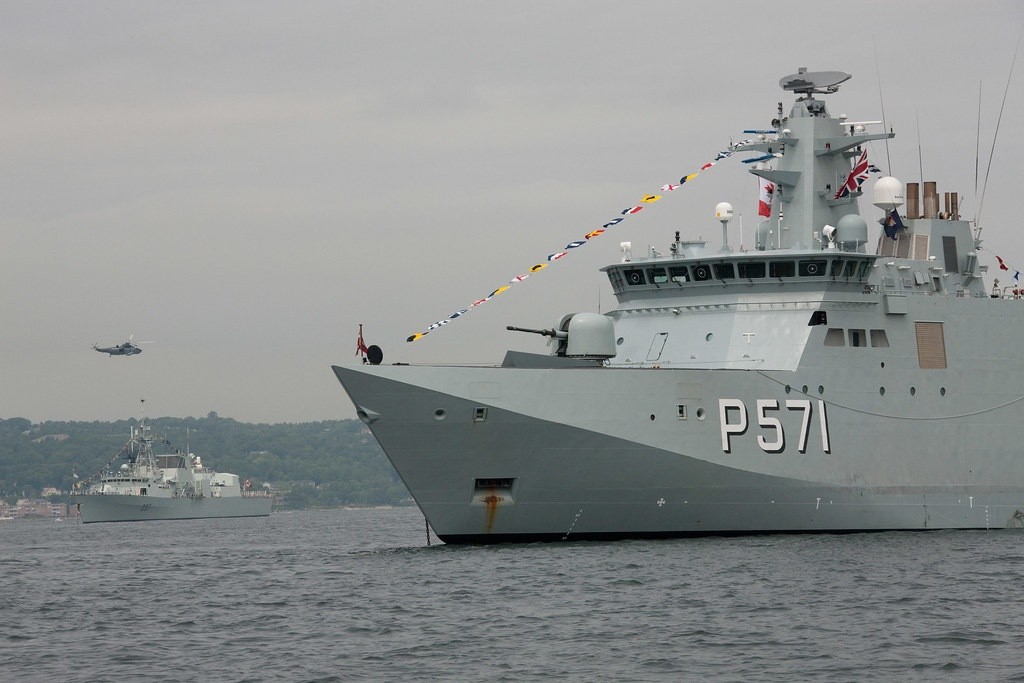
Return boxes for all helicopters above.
[90,334,154,357]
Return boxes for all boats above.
[389,495,418,507]
[68,420,275,524]
[329,61,1024,545]
[53,518,64,522]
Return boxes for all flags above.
[407,286,510,343]
[563,194,663,248]
[509,253,567,283]
[868,165,881,173]
[835,148,869,199]
[661,139,749,192]
[884,210,903,237]
[996,256,1008,271]
[356,329,369,355]
[759,177,775,218]
